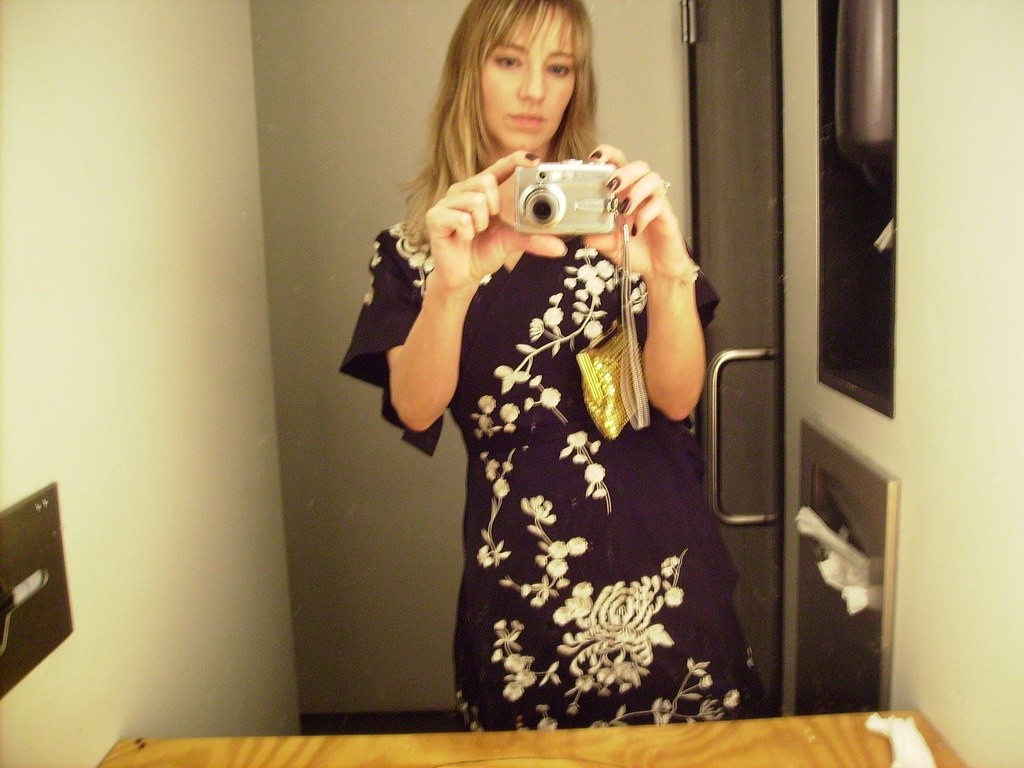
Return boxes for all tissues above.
[831,1,897,258]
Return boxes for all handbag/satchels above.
[575,321,650,443]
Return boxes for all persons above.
[337,0,775,733]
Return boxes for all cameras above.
[514,158,617,235]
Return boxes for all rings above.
[655,180,672,198]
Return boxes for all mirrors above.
[0,0,901,768]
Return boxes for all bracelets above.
[646,265,701,287]
